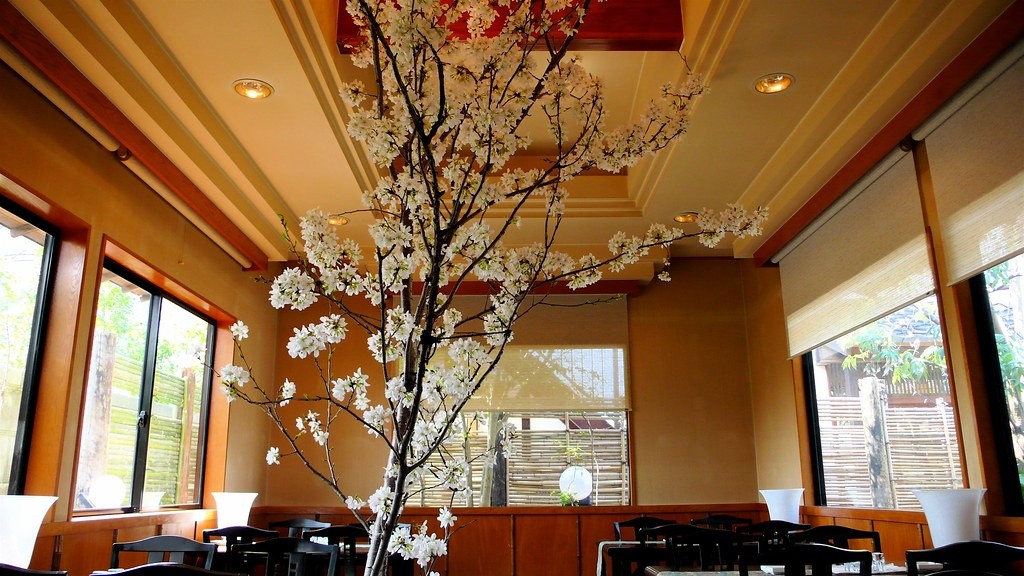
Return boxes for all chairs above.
[110,534,217,571]
[614,514,883,576]
[905,541,1024,576]
[202,518,412,576]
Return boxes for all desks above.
[598,538,833,576]
[643,561,1024,576]
[188,542,415,576]
